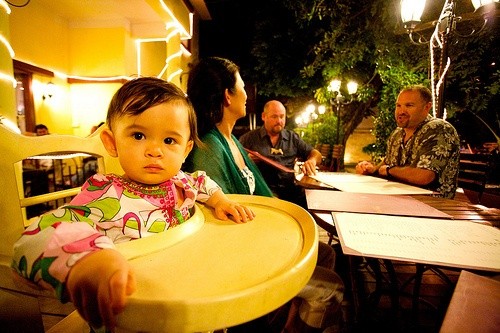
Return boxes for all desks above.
[295,171,500,320]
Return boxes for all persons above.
[355,84,460,203]
[181,57,345,333]
[90,122,105,137]
[238,100,322,209]
[10,76,255,328]
[34,124,49,137]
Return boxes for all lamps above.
[331,79,359,105]
[400,0,496,45]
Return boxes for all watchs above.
[386,163,397,179]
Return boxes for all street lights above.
[330,79,358,145]
[306,105,325,132]
[400,0,500,119]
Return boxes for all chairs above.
[0,123,319,333]
[438,270,500,333]
[458,154,493,205]
